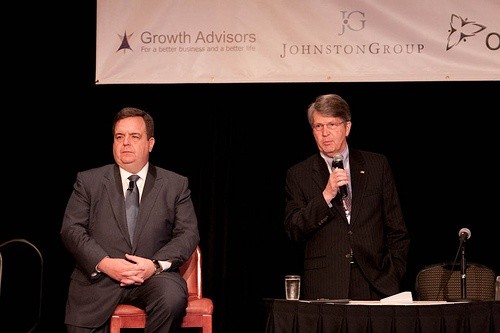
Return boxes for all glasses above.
[312,122,345,131]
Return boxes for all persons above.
[282,95,411,301]
[59,107,199,333]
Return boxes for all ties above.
[332,161,352,216]
[125,175,141,248]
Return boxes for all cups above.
[284,275,301,301]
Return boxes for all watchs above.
[152,259,162,275]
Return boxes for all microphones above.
[459,228,471,242]
[333,153,348,198]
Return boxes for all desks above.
[266,298,500,333]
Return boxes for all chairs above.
[415,262,496,301]
[110,244,213,333]
[0,239,43,333]
